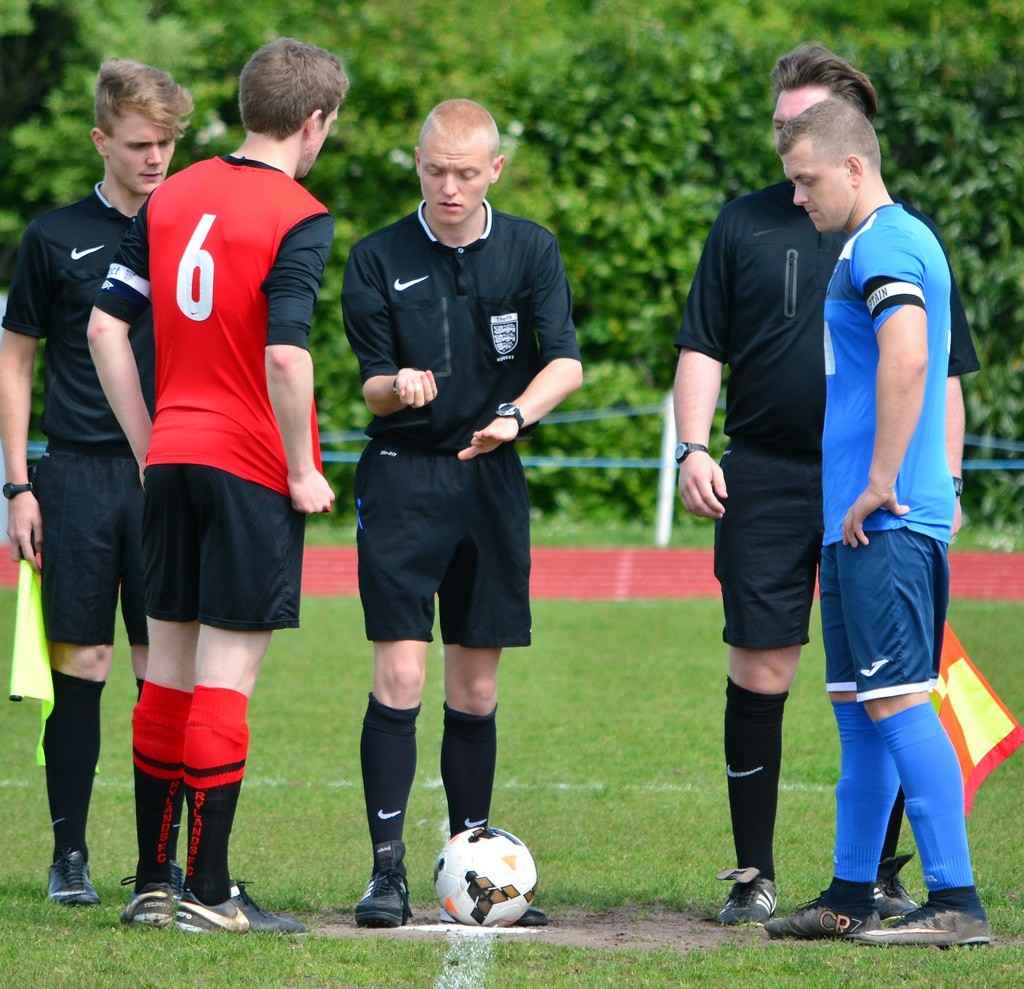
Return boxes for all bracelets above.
[393,378,399,395]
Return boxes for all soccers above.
[432,827,538,928]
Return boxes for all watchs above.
[494,402,523,429]
[3,482,32,500]
[674,442,709,463]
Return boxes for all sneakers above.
[47,846,100,907]
[119,876,182,928]
[174,876,308,938]
[764,889,882,942]
[355,841,413,927]
[874,851,918,919]
[513,909,548,926]
[715,866,776,925]
[854,900,988,947]
[169,860,184,904]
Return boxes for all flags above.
[929,618,1024,820]
[9,559,101,778]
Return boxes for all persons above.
[0,57,191,906]
[762,98,992,949]
[337,100,584,927]
[670,44,986,921]
[81,38,352,937]
